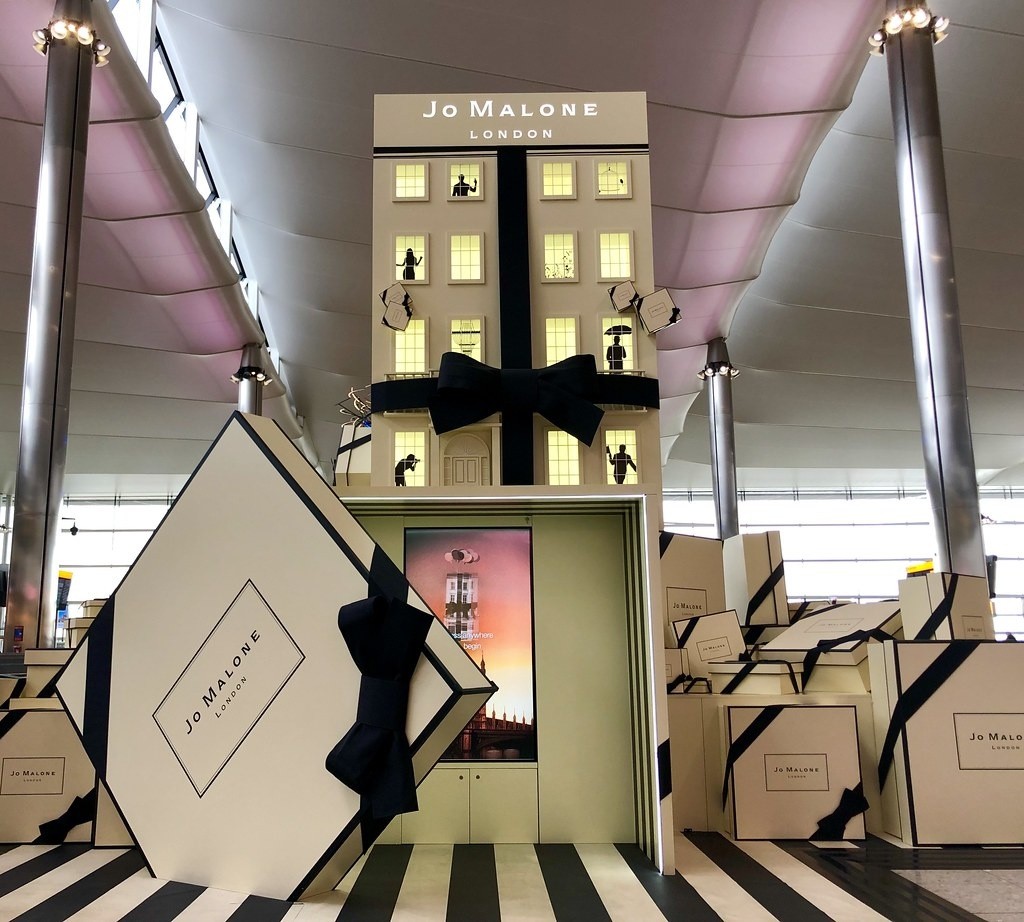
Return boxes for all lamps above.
[31,16,114,73]
[225,361,277,391]
[694,358,742,381]
[867,7,951,56]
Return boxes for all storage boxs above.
[378,280,413,311]
[381,300,413,334]
[628,288,684,336]
[607,279,641,312]
[0,408,1024,901]
